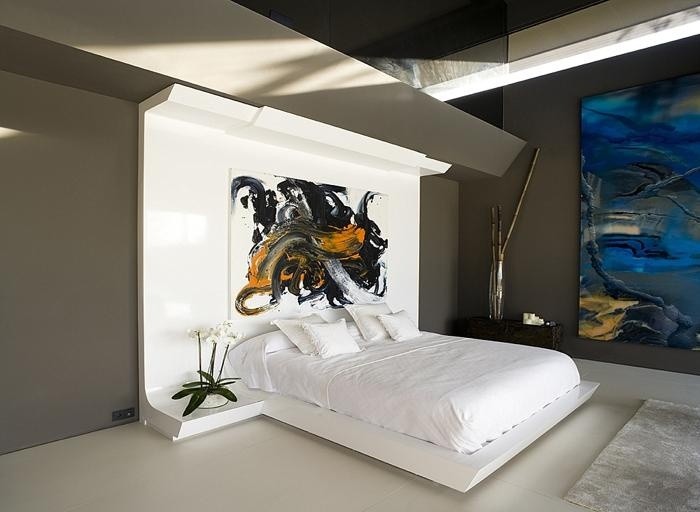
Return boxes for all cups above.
[523,313,543,324]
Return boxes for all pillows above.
[274,302,421,360]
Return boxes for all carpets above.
[560,400,700,512]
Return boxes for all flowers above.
[171,320,241,417]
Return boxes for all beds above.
[240,326,600,494]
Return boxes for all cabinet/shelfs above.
[465,315,562,351]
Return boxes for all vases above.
[487,261,506,321]
[198,393,228,409]
